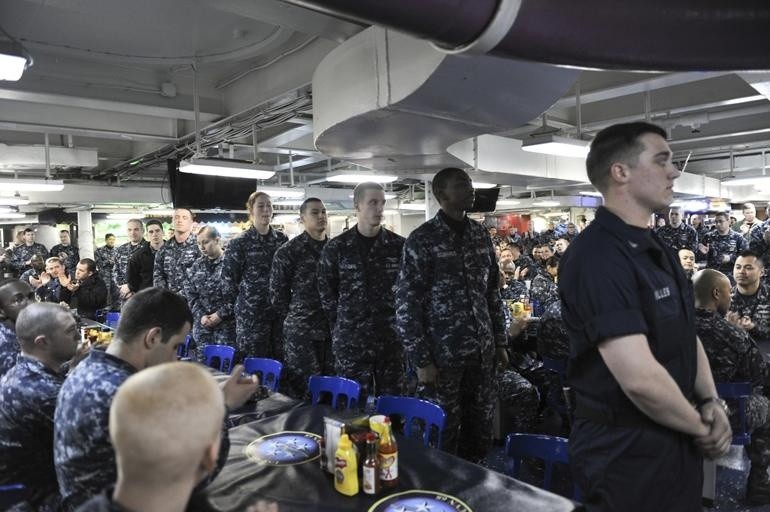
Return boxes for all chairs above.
[701,377,756,508]
[1,484,27,505]
[177,310,581,501]
[107,313,121,328]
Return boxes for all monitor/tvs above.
[467,187,501,212]
[166,158,258,215]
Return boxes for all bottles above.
[512,295,531,321]
[83,328,112,347]
[330,414,399,496]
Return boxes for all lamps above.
[320,154,399,186]
[177,59,277,180]
[0,29,35,83]
[0,132,65,191]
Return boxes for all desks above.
[202,404,577,512]
[81,318,117,334]
